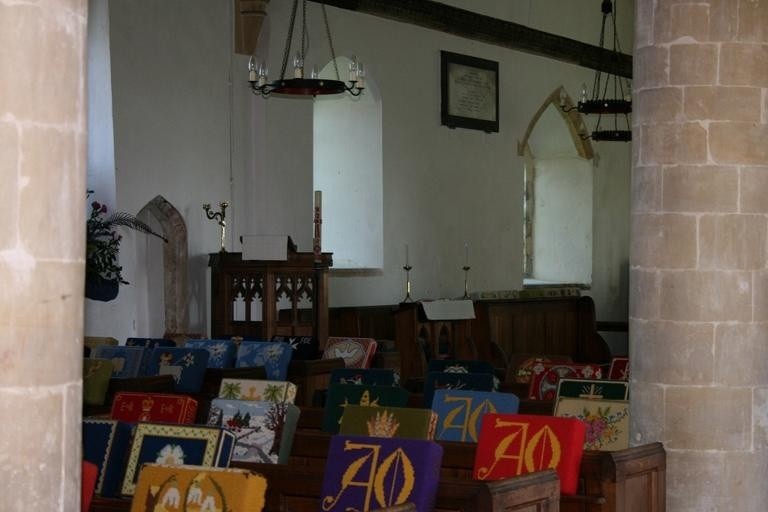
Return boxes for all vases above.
[85,272,119,302]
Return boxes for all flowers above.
[87,189,168,285]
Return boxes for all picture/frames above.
[440,50,499,133]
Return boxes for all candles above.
[315,191,322,238]
[406,241,409,266]
[465,242,469,266]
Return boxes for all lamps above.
[248,0,367,99]
[560,0,632,114]
[578,0,632,141]
